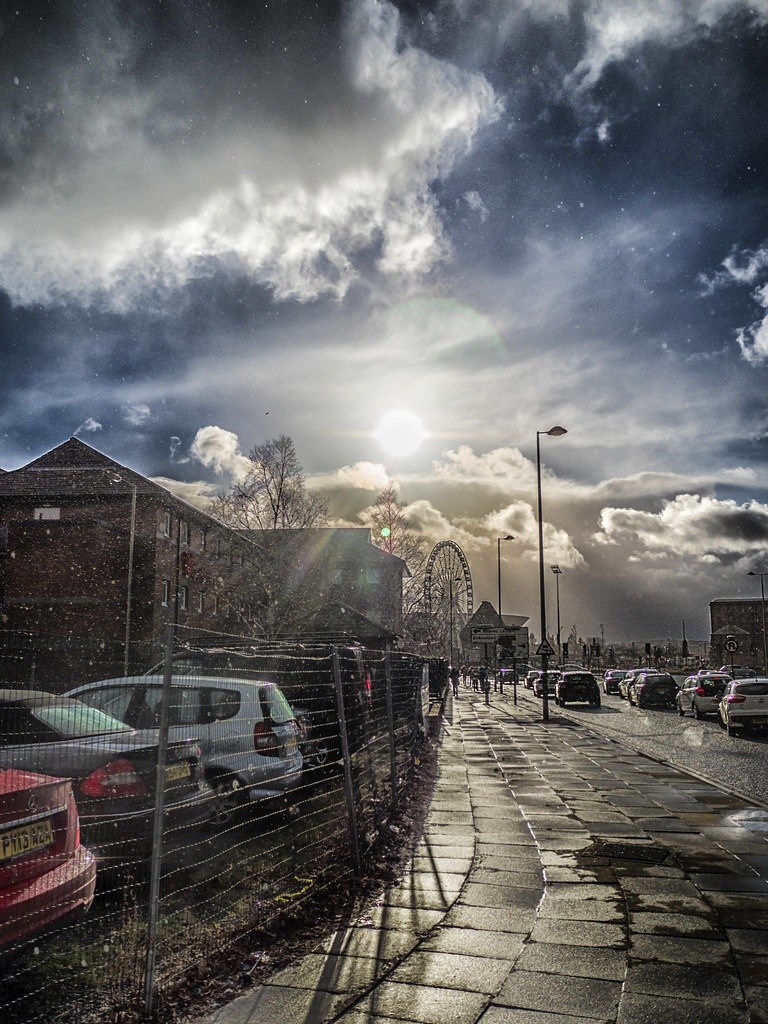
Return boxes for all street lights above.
[746,571,768,678]
[107,472,137,675]
[449,578,463,666]
[549,565,564,665]
[498,535,515,692]
[536,427,568,721]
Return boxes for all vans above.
[142,645,376,776]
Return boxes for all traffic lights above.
[563,643,569,659]
[583,644,587,657]
[609,649,612,659]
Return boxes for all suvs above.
[509,663,539,678]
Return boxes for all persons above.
[461,665,488,691]
[449,668,460,697]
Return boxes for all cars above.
[629,672,681,710]
[496,668,519,684]
[558,663,587,672]
[532,671,562,697]
[618,669,660,700]
[58,675,305,831]
[0,687,215,872]
[674,674,734,720]
[552,670,601,708]
[524,671,543,689]
[603,669,628,694]
[720,664,757,678]
[698,670,727,685]
[0,768,98,969]
[717,678,768,736]
